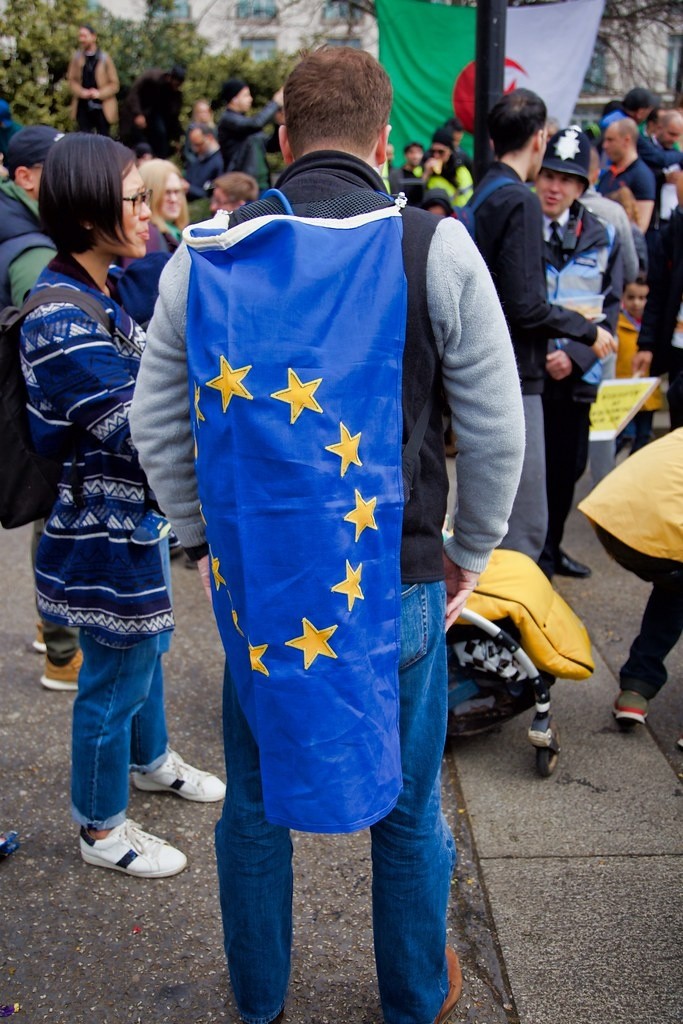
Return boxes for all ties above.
[547,220,562,254]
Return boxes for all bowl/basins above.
[549,294,605,321]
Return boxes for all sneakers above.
[79,820,188,878]
[613,690,650,724]
[39,647,85,691]
[32,621,47,653]
[134,748,226,802]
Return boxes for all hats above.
[431,127,456,152]
[220,80,246,105]
[0,99,11,127]
[3,125,67,182]
[541,128,591,192]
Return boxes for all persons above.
[210,80,285,189]
[179,99,224,201]
[68,26,120,136]
[209,173,259,217]
[1,97,84,690]
[119,156,188,269]
[120,66,185,159]
[576,426,683,749]
[467,86,683,581]
[420,187,459,458]
[21,131,227,877]
[388,117,475,207]
[127,42,526,1024]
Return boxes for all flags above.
[376,0,605,168]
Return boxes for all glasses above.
[211,196,240,207]
[429,148,445,154]
[124,188,154,216]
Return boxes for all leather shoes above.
[553,549,592,578]
[432,946,461,1024]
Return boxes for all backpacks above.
[0,287,112,529]
[448,171,521,239]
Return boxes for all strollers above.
[442,520,564,778]
